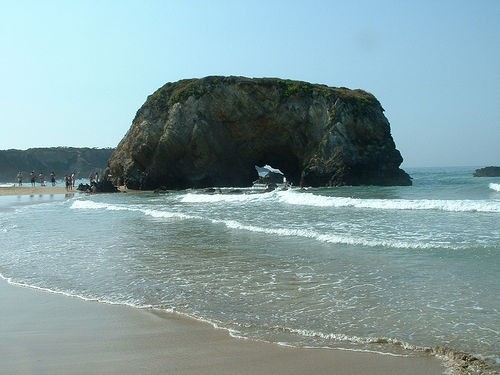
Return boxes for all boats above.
[251,163,290,195]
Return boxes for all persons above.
[17,172,23,185]
[50,171,55,184]
[39,172,44,185]
[64,175,69,190]
[31,171,36,186]
[89,173,93,185]
[95,171,99,184]
[101,173,128,189]
[71,172,76,187]
[68,175,72,190]
